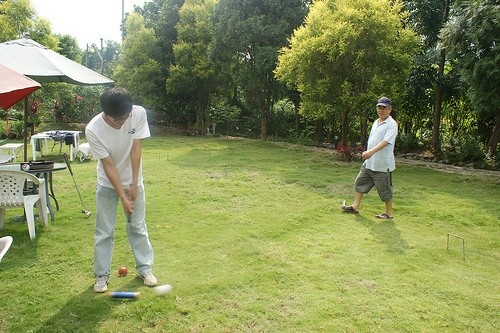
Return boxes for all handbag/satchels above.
[19,160,55,170]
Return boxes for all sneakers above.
[139,273,158,287]
[93,277,108,293]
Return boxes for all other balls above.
[119,266,127,277]
[153,284,172,296]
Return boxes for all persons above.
[86,87,157,291]
[341,97,398,219]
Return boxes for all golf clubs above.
[343,159,367,206]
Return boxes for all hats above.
[376,97,392,107]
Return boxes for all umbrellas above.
[0,32,115,162]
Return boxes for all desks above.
[21,162,67,218]
[0,142,25,161]
[30,129,82,161]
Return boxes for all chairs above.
[0,168,49,240]
[0,234,14,262]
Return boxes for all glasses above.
[377,107,386,111]
[113,113,133,123]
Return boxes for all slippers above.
[375,212,394,219]
[341,205,359,214]
